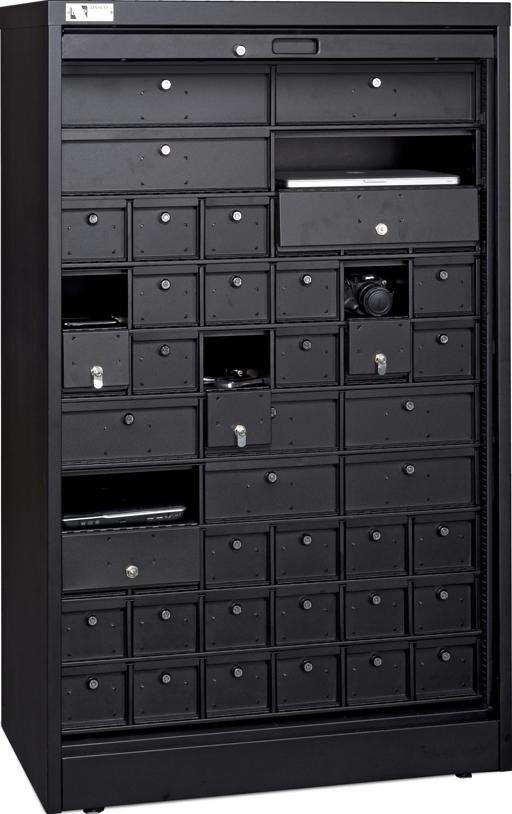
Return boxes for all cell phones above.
[62,317,119,328]
[214,376,263,389]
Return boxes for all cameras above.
[343,273,395,317]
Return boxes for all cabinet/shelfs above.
[0,0,512,814]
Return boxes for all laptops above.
[274,167,462,189]
[61,501,188,530]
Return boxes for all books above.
[284,170,461,188]
